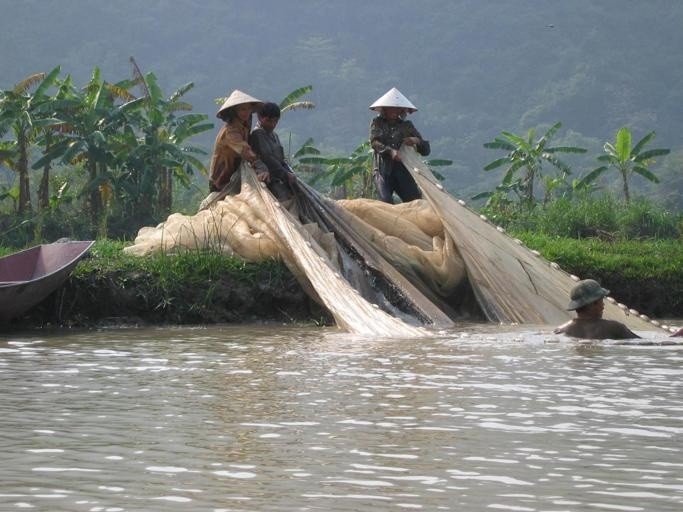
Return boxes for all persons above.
[369,87,431,205]
[250,102,281,158]
[553,279,643,340]
[208,89,271,193]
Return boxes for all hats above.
[566,277,611,312]
[215,88,264,120]
[367,86,418,114]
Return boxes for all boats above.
[0,239,97,328]
